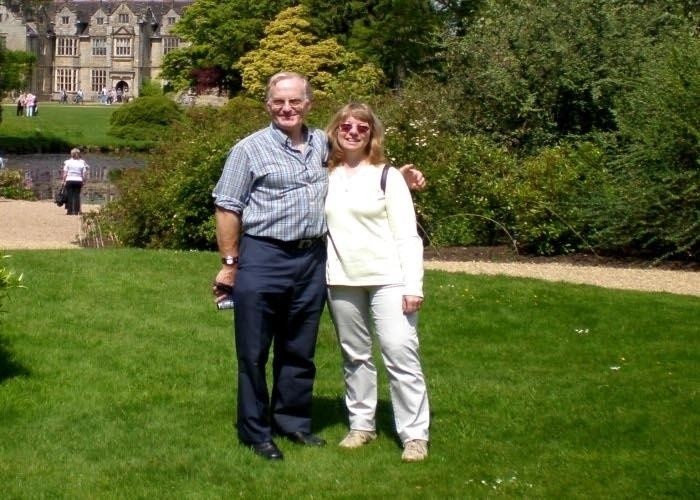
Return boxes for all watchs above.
[221,256,239,265]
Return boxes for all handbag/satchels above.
[54,183,67,207]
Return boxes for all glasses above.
[270,98,304,109]
[340,123,370,133]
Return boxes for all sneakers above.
[401,439,429,462]
[339,430,378,449]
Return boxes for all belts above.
[243,230,329,249]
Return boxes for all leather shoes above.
[284,431,325,447]
[242,440,283,459]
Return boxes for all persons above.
[60,83,130,106]
[60,147,87,216]
[16,91,40,118]
[322,100,432,465]
[212,71,427,464]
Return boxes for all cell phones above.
[217,297,234,309]
